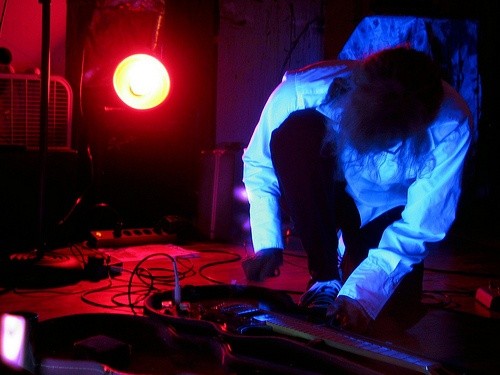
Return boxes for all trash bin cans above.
[194,147,233,241]
[233,149,256,246]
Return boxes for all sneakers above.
[305,271,343,324]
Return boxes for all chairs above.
[0,72,95,234]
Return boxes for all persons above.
[337,15,484,143]
[239,45,475,333]
